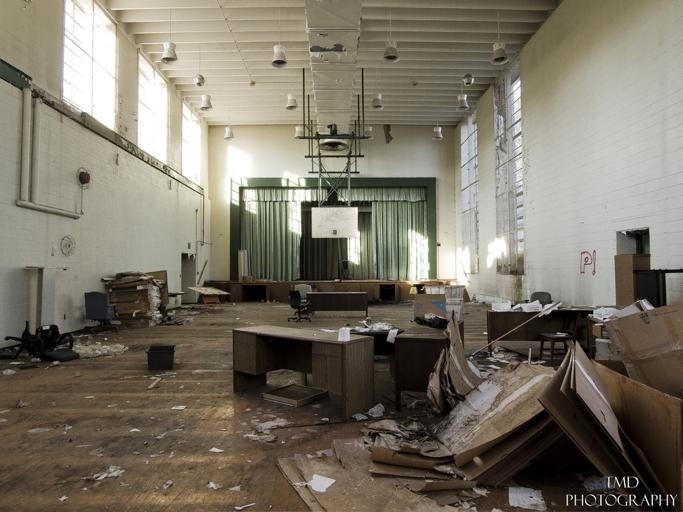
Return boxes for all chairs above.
[286,284,313,323]
[530,292,552,306]
[84,292,118,332]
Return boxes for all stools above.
[538,329,576,365]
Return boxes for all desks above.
[231,319,465,422]
[485,303,602,354]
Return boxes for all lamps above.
[159,9,234,140]
[270,5,307,141]
[432,10,509,141]
[360,11,400,143]
